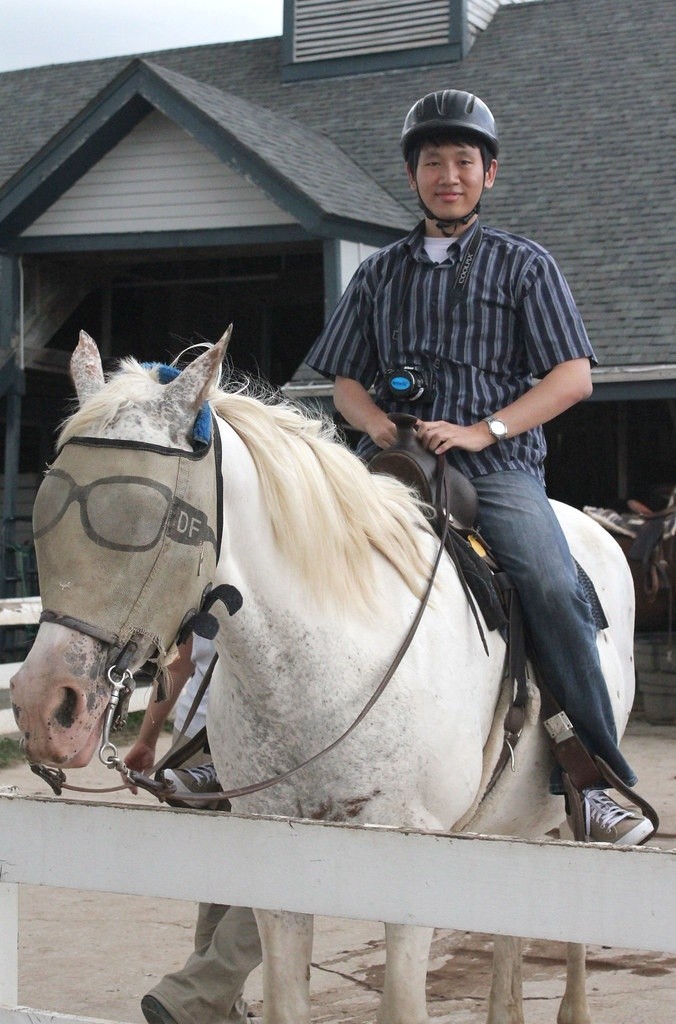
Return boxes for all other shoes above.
[141,995,179,1024]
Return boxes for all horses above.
[10,323,637,1024]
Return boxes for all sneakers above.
[163,760,222,810]
[565,787,654,845]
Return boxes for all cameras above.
[380,365,437,405]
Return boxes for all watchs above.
[482,416,507,442]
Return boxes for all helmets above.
[399,89,499,156]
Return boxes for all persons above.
[159,88,656,847]
[122,623,262,1024]
